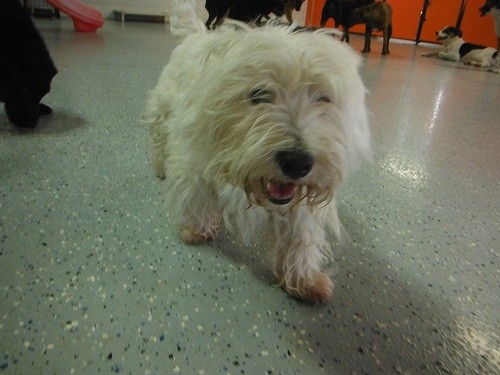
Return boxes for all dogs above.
[264,0,305,25]
[421,26,500,68]
[142,0,373,305]
[478,0,500,73]
[320,0,375,43]
[205,0,284,30]
[345,0,393,55]
